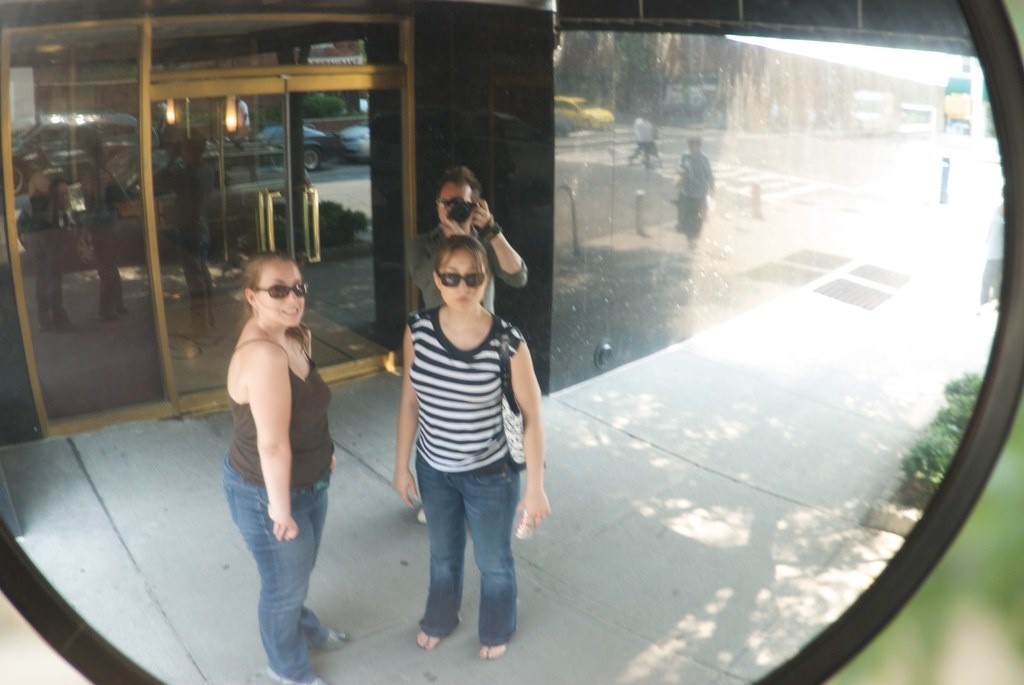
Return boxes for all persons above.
[627,110,660,168]
[17,125,216,334]
[226,252,348,685]
[406,166,527,526]
[670,136,715,249]
[392,234,549,659]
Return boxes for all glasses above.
[252,282,310,298]
[435,197,465,208]
[432,267,484,287]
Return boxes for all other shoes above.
[265,664,326,685]
[307,626,346,651]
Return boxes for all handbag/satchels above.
[499,321,526,464]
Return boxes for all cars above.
[239,123,371,171]
[553,96,614,136]
[12,113,160,194]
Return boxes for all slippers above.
[478,632,517,659]
[415,626,459,650]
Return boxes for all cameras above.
[445,196,476,223]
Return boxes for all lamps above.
[165,99,176,126]
[226,96,237,132]
